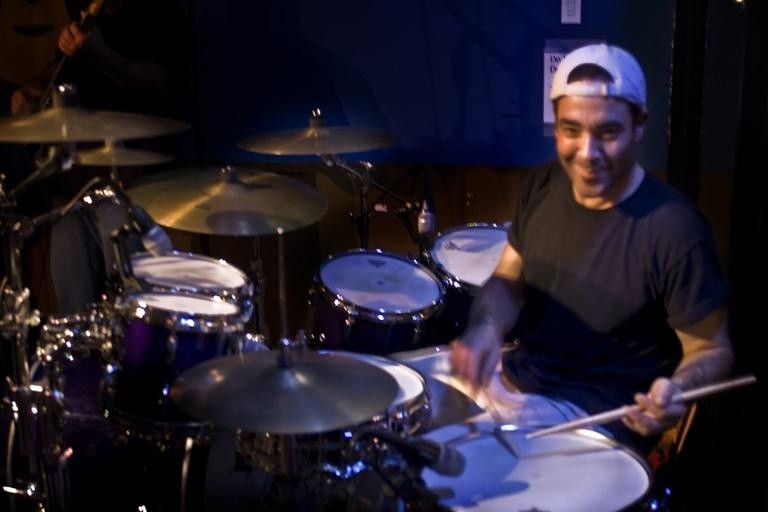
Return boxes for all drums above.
[119,250,257,337]
[418,424,658,510]
[284,351,432,509]
[301,247,448,358]
[100,292,247,446]
[17,178,173,425]
[423,221,523,343]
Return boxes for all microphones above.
[416,168,436,235]
[371,424,465,476]
[102,173,173,259]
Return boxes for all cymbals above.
[238,124,399,156]
[66,149,174,167]
[170,346,399,437]
[122,167,330,237]
[0,108,194,143]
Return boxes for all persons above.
[450,44,734,459]
[10,0,203,211]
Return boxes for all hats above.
[548,41,649,113]
